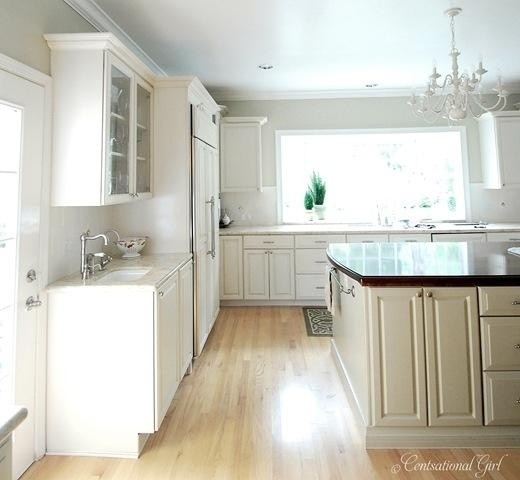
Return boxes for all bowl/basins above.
[113,236,149,258]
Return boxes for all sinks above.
[95,268,151,281]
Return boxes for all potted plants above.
[304,192,313,221]
[307,169,326,220]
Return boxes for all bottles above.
[222,214,230,225]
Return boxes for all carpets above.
[304,305,334,337]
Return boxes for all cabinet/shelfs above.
[478,111,520,190]
[478,275,520,449]
[368,276,482,449]
[220,232,520,307]
[220,117,268,193]
[112,75,220,360]
[44,33,161,207]
[47,258,194,459]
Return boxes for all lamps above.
[407,8,511,128]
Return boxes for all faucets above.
[79,233,108,280]
[99,229,120,271]
